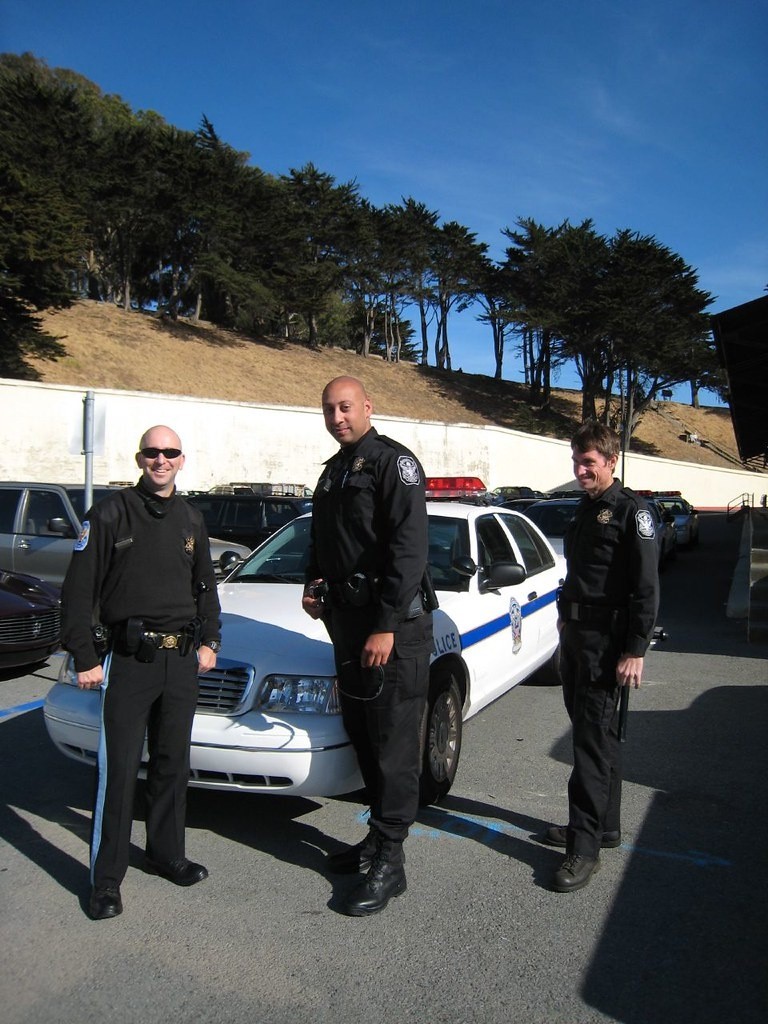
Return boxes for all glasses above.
[139,448,183,459]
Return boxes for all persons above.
[61,425,224,921]
[303,375,440,916]
[544,418,660,891]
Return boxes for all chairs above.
[431,525,495,580]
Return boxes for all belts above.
[144,632,184,649]
[565,601,625,622]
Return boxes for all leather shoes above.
[327,835,406,873]
[142,854,208,886]
[343,860,408,917]
[545,824,622,848]
[553,854,601,893]
[87,887,122,920]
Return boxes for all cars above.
[43,477,568,805]
[0,482,699,669]
